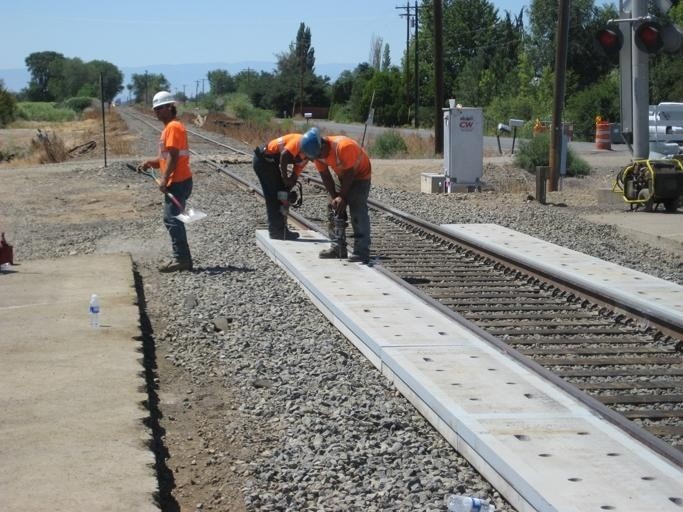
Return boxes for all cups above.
[449,99,455,108]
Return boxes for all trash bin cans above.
[611,123,621,144]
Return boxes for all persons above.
[135,91,194,272]
[300,128,371,262]
[252,133,318,241]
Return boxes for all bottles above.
[88,295,100,329]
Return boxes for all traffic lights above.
[633,22,664,53]
[596,25,624,54]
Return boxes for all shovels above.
[140,166,207,224]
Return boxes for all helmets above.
[300,130,323,160]
[152,91,176,109]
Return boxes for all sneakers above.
[348,253,370,262]
[318,246,348,259]
[158,259,174,272]
[168,256,193,271]
[270,228,301,240]
